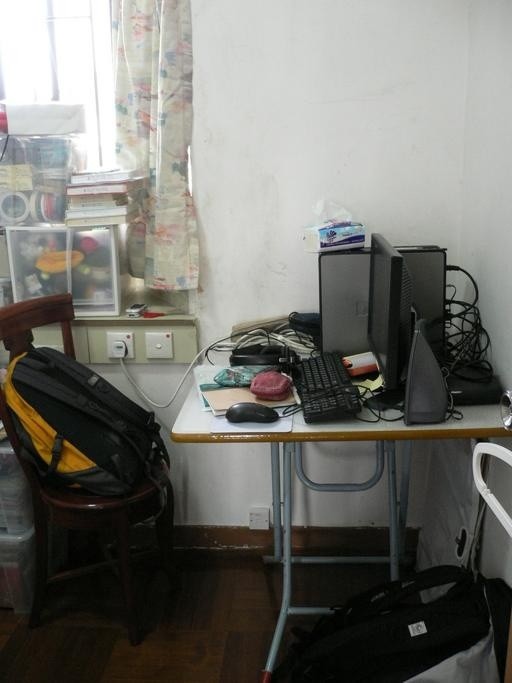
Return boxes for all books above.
[204,383,296,417]
[199,383,228,412]
[66,167,151,227]
[335,349,379,376]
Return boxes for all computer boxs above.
[317,243,447,361]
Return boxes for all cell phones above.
[125,303,148,318]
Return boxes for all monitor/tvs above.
[362,232,414,411]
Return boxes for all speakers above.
[404,318,448,425]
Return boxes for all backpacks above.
[1,345,171,496]
[284,560,511,683]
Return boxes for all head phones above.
[229,344,298,375]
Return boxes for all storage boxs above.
[5,223,126,319]
[0,440,41,620]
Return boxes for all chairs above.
[0,295,181,646]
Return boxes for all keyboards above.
[294,350,362,422]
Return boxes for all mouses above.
[225,402,279,422]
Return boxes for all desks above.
[167,371,512,683]
